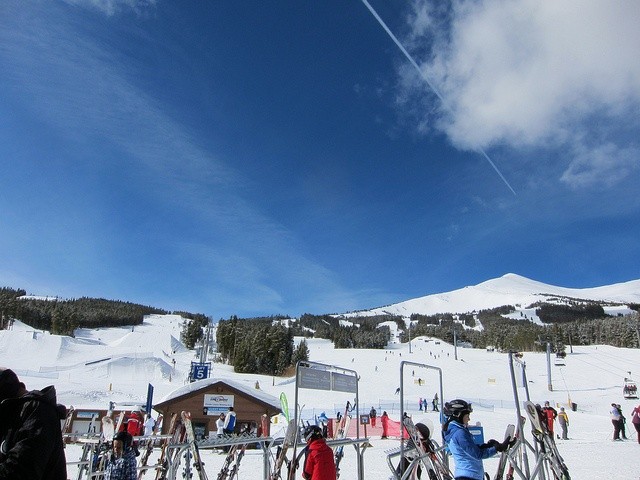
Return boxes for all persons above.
[610,402,623,441]
[631,405,640,444]
[370,406,376,427]
[542,400,557,440]
[302,425,337,480]
[224,407,236,434]
[423,399,428,413]
[616,404,627,440]
[215,413,225,438]
[432,392,440,412]
[441,399,516,480]
[0,367,68,480]
[143,413,156,436]
[104,431,138,480]
[125,407,143,435]
[558,406,570,440]
[380,411,389,440]
[419,397,423,411]
[394,387,400,395]
[418,378,422,386]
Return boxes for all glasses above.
[450,403,472,412]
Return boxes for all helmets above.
[302,425,324,442]
[444,399,473,419]
[113,431,132,451]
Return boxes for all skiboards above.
[228,443,249,480]
[217,430,245,480]
[535,402,571,480]
[137,415,163,480]
[271,418,295,480]
[493,424,516,480]
[159,421,182,480]
[506,416,527,480]
[181,410,209,480]
[182,449,193,480]
[402,415,437,480]
[523,401,570,480]
[75,413,96,480]
[155,413,178,480]
[334,415,351,480]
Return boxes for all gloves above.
[496,435,517,452]
[485,438,500,447]
